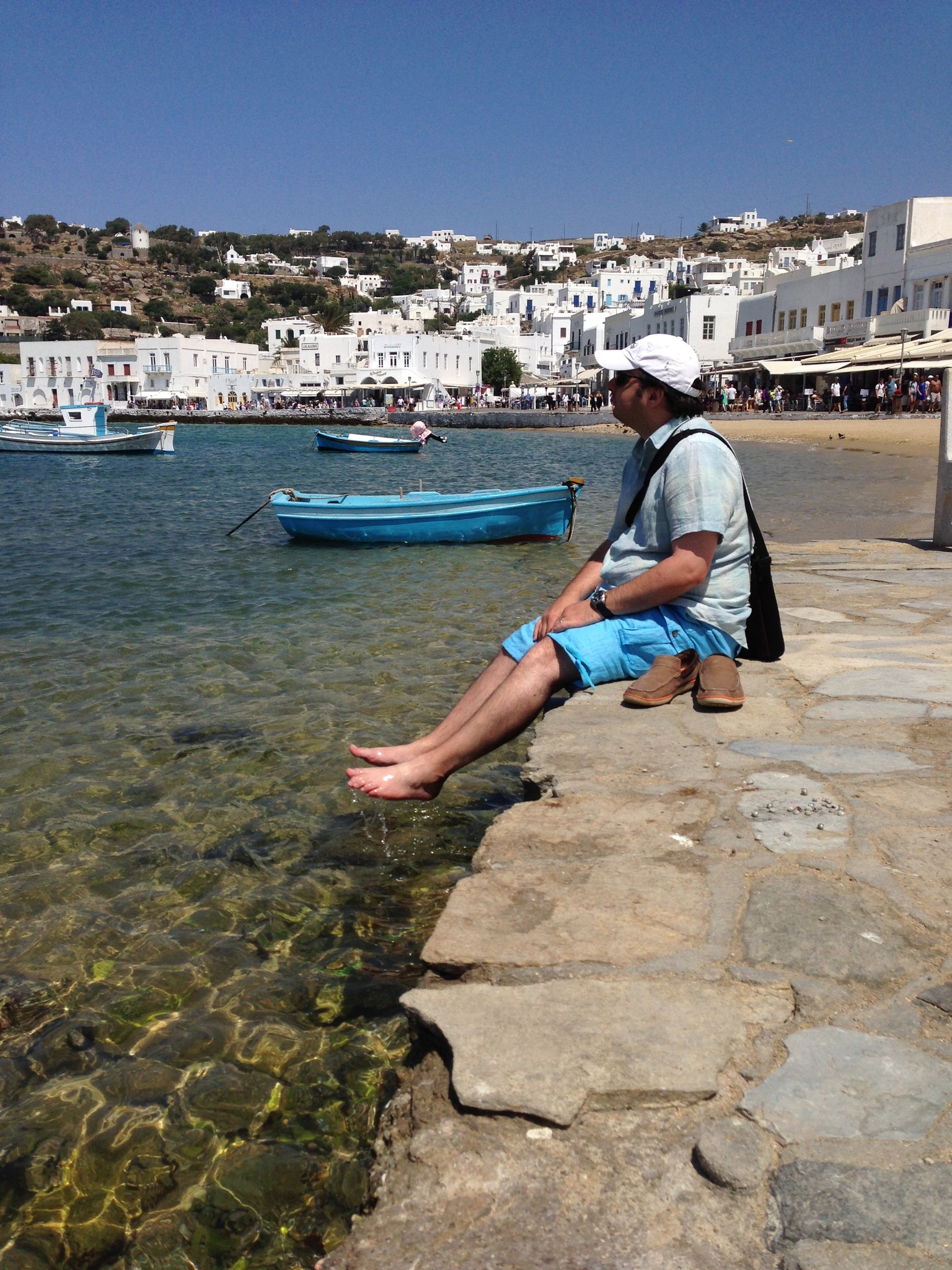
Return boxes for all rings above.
[559,618,563,623]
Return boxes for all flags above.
[91,368,103,378]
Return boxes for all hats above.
[777,385,782,389]
[399,396,401,398]
[594,333,704,399]
[927,375,934,379]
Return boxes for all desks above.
[747,399,754,410]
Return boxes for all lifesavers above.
[84,402,104,405]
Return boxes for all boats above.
[266,476,586,542]
[312,421,448,451]
[0,399,181,455]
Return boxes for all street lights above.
[229,384,234,411]
[478,369,481,416]
[407,376,412,401]
[573,362,582,412]
[139,385,143,409]
[711,359,720,399]
[897,327,910,393]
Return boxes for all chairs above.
[485,399,590,409]
[810,396,823,411]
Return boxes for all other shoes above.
[842,409,848,412]
[696,651,745,708]
[768,411,773,413]
[622,648,702,707]
[775,411,778,413]
[780,410,783,413]
[754,411,760,413]
[928,408,937,414]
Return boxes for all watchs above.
[590,590,615,618]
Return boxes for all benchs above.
[787,398,804,410]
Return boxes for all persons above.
[347,334,754,801]
[874,372,942,414]
[350,397,375,407]
[223,401,231,410]
[275,399,337,409]
[466,394,484,409]
[398,396,416,412]
[235,401,266,410]
[706,376,852,415]
[547,391,614,412]
[185,400,202,410]
[500,394,531,410]
[438,394,462,411]
[127,400,175,410]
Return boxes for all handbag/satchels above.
[602,402,604,406]
[555,405,558,409]
[737,541,785,662]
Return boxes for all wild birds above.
[582,428,584,430]
[838,433,846,439]
[572,427,576,430]
[829,434,833,440]
[614,424,638,434]
[606,425,611,427]
